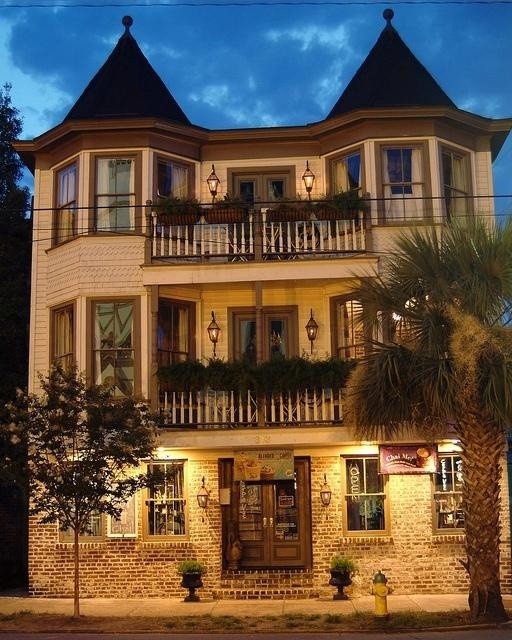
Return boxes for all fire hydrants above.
[366,570,395,620]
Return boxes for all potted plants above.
[175,561,209,603]
[325,556,358,601]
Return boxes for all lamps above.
[206,311,221,360]
[320,472,334,506]
[304,308,319,355]
[300,159,317,194]
[195,476,210,508]
[204,162,221,202]
